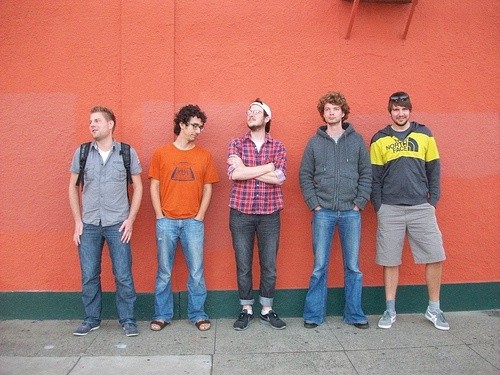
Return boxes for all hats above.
[250,102,271,133]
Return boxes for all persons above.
[369,91,451,331]
[147,103,221,331]
[298,91,371,329]
[68,107,142,337]
[226,97,286,331]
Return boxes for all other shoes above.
[354,322,370,328]
[303,320,318,328]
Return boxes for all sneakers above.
[233,309,255,331]
[425,305,450,330]
[377,311,397,328]
[73,323,100,336]
[123,323,139,337]
[260,310,286,329]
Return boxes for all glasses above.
[188,123,203,132]
[246,110,263,115]
[390,94,409,103]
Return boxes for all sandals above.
[195,320,211,331]
[150,321,170,331]
